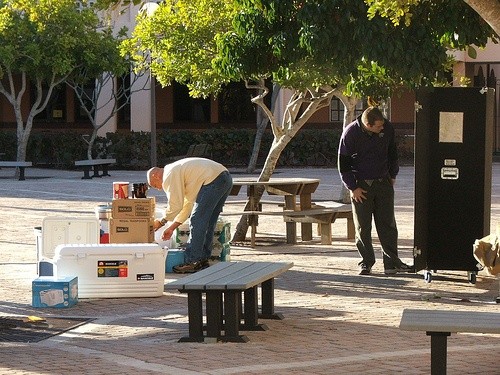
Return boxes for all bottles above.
[131,181,147,198]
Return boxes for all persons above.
[338,107,414,274]
[147,158,233,274]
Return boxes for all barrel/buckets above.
[95,203,112,221]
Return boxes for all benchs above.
[399,308,500,375]
[0,161,32,181]
[164,261,294,343]
[218,198,355,244]
[75,159,116,180]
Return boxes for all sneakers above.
[358,263,372,275]
[172,259,209,274]
[385,264,413,276]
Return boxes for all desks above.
[229,176,320,245]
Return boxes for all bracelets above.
[160,218,167,225]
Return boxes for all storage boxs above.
[165,249,184,273]
[109,216,155,244]
[52,243,165,298]
[177,243,230,266]
[176,221,231,244]
[111,197,155,219]
[32,275,79,308]
